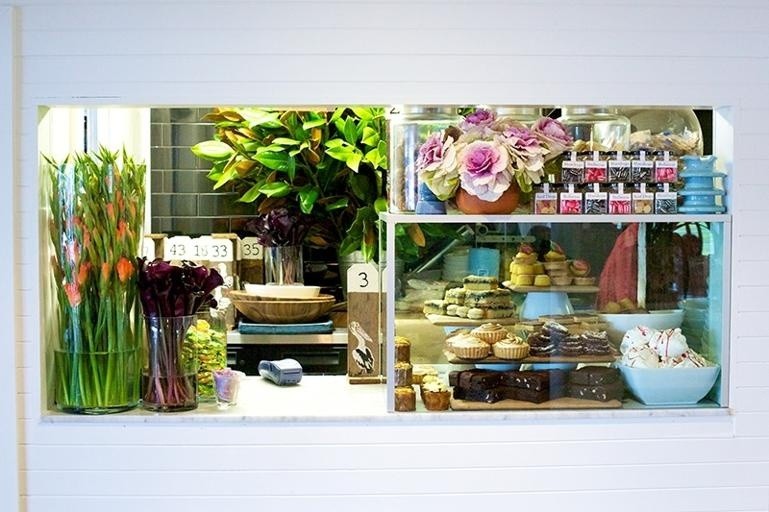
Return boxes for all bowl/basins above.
[616,363,722,406]
[598,309,687,353]
[229,284,336,324]
[441,246,468,282]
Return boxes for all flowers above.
[244,207,309,283]
[181,318,228,398]
[133,256,225,405]
[416,108,573,202]
[36,142,147,405]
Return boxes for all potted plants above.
[190,106,467,303]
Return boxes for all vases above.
[263,246,303,284]
[41,163,147,416]
[142,311,228,414]
[454,175,520,215]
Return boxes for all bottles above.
[556,106,629,154]
[384,106,462,214]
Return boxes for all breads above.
[601,298,649,314]
[510,239,596,286]
[445,324,530,361]
[395,336,416,411]
[413,364,451,411]
[448,368,623,404]
[620,326,706,369]
[515,312,611,357]
[423,272,519,323]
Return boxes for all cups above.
[213,370,247,410]
[469,248,500,284]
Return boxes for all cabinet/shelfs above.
[380,106,730,412]
